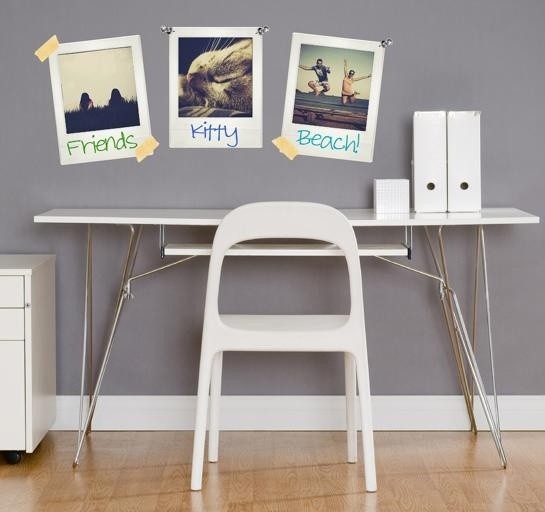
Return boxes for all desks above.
[34,206,541,474]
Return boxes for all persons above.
[108,89,125,106]
[342,58,372,105]
[298,58,331,97]
[79,92,94,110]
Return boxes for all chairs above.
[190,201,379,491]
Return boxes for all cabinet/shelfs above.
[0,254,56,466]
